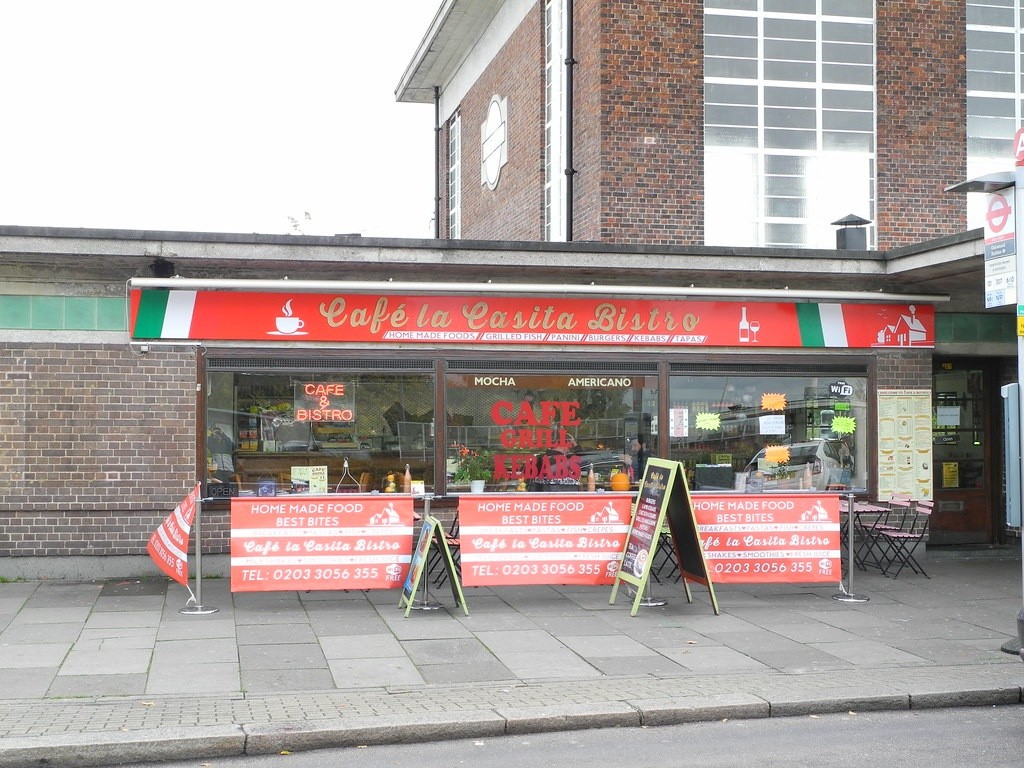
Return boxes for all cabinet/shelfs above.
[671,397,791,444]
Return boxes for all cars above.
[279,440,319,452]
[529,451,635,491]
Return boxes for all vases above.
[471,480,485,492]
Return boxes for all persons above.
[524,433,585,492]
[630,435,657,482]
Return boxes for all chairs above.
[360,472,369,493]
[631,504,680,580]
[429,506,480,589]
[861,493,935,580]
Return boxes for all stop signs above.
[982,186,1018,308]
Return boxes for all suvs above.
[741,437,852,491]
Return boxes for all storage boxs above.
[256,476,277,497]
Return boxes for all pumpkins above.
[610,471,630,491]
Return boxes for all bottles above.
[403,464,412,493]
[587,463,595,491]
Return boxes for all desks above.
[840,500,893,579]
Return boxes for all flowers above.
[453,445,495,480]
